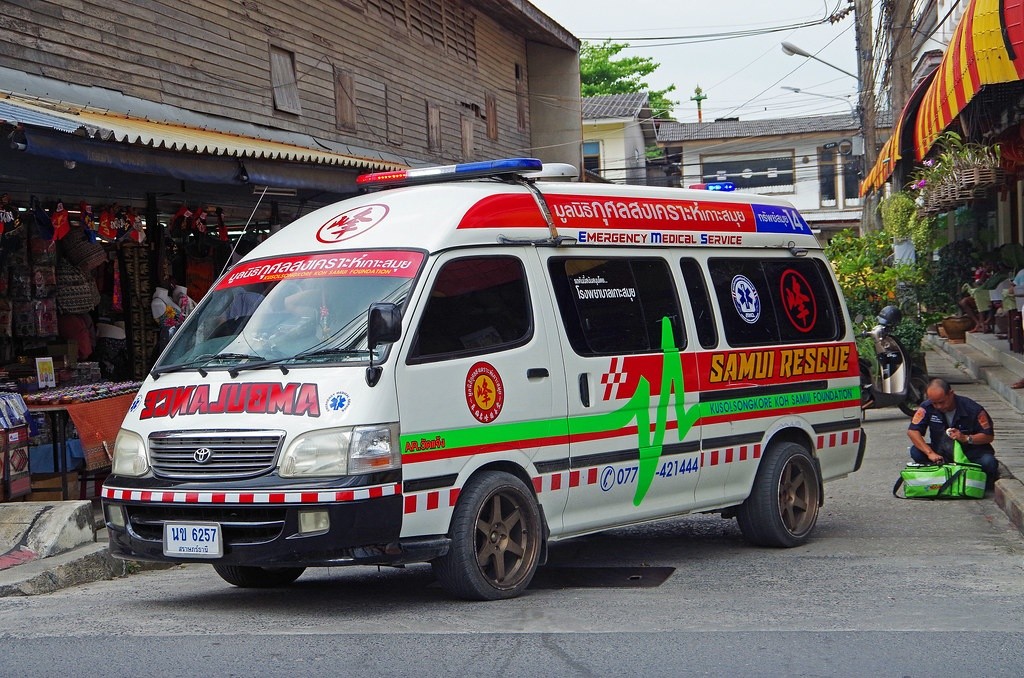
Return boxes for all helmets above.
[876,305,902,325]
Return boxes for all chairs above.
[957,270,1024,353]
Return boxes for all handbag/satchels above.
[28,197,56,266]
[893,439,987,500]
[63,228,107,273]
[56,245,101,318]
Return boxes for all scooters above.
[855,306,929,417]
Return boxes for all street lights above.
[781,42,882,233]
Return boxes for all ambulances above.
[102,159,867,601]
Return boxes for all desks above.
[22,379,144,511]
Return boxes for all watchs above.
[967,434,973,444]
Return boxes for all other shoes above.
[1010,378,1024,388]
[971,323,991,334]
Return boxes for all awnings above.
[0,90,414,189]
[859,0,1023,200]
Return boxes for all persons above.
[283,279,334,342]
[1010,304,1024,390]
[959,248,1024,346]
[907,377,999,491]
[149,284,199,357]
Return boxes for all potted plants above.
[941,299,970,344]
[921,308,951,338]
